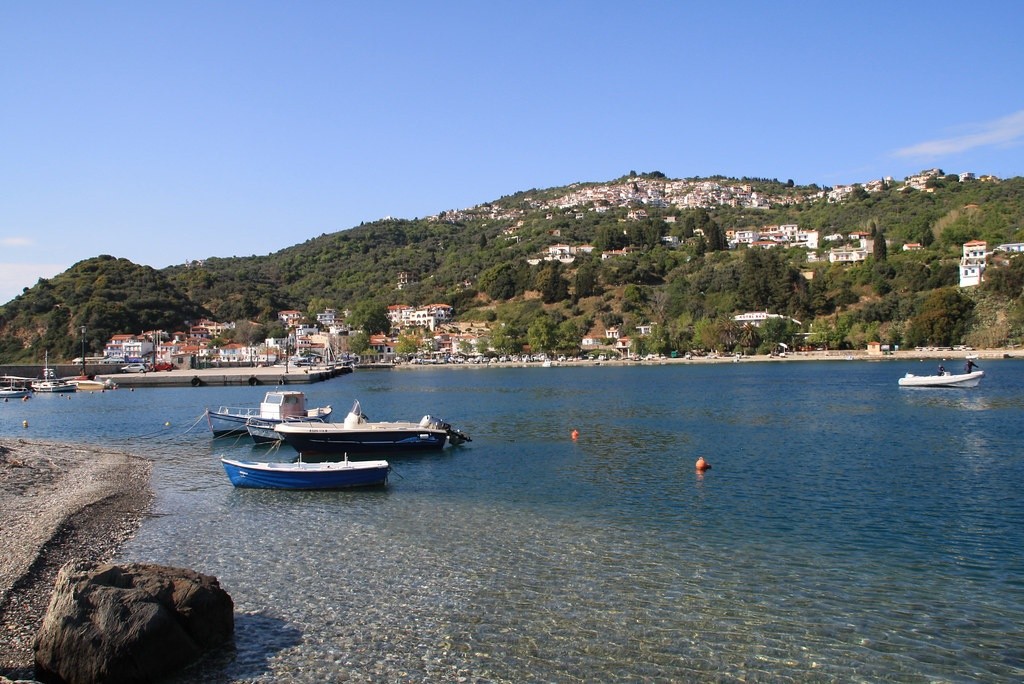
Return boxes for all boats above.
[206,391,333,437]
[276,398,472,457]
[0,387,28,399]
[899,370,986,389]
[246,419,282,444]
[30,350,76,392]
[220,457,389,491]
[65,380,119,391]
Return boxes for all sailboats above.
[328,341,354,368]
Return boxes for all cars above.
[295,360,316,368]
[411,353,667,361]
[953,345,962,350]
[394,356,401,364]
[151,362,173,371]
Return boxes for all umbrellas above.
[780,343,787,352]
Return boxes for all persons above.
[965,359,979,373]
[937,364,945,376]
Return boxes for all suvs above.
[121,363,148,373]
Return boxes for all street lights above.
[151,336,155,371]
[80,326,88,375]
[283,322,291,373]
[124,352,127,363]
